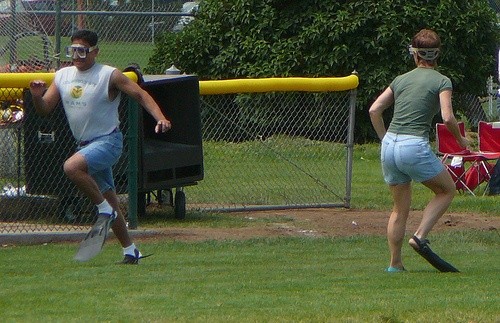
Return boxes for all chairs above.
[435,121,500,197]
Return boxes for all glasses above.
[65,43,99,59]
[407,44,439,60]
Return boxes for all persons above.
[30,30,172,265]
[369,29,468,273]
